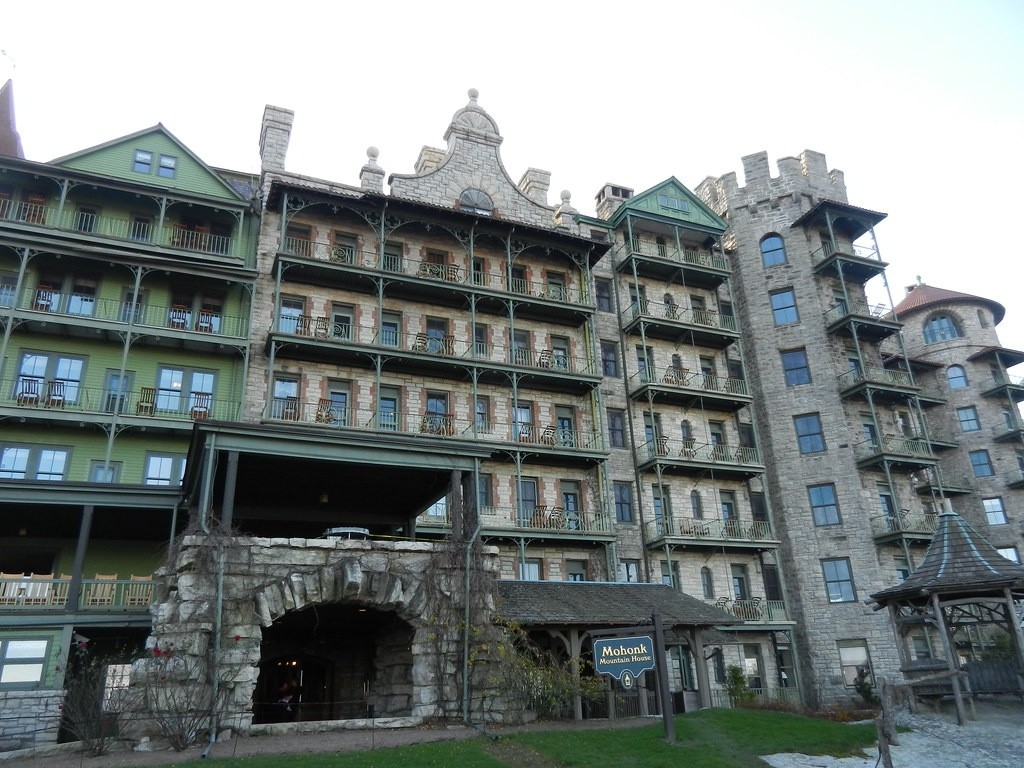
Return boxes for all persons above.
[278,672,302,722]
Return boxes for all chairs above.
[330,242,346,263]
[0,572,25,605]
[530,505,547,527]
[515,346,529,365]
[416,259,431,277]
[874,433,894,453]
[0,192,46,224]
[169,304,187,329]
[519,422,534,443]
[682,246,709,265]
[169,223,188,248]
[854,359,909,385]
[536,348,553,368]
[191,225,211,251]
[195,308,213,333]
[23,572,54,605]
[124,574,152,605]
[548,505,565,528]
[191,392,209,420]
[296,314,312,336]
[50,573,85,605]
[411,331,430,352]
[729,596,748,616]
[421,411,437,433]
[315,397,332,424]
[437,412,454,435]
[849,296,887,317]
[889,508,939,531]
[715,596,730,609]
[32,284,53,312]
[314,316,330,338]
[657,435,752,465]
[44,380,65,410]
[747,596,761,619]
[438,334,455,355]
[662,365,737,394]
[86,573,118,605]
[444,263,460,281]
[16,378,40,408]
[282,395,301,421]
[679,515,766,540]
[135,387,156,417]
[538,424,558,445]
[633,296,718,327]
